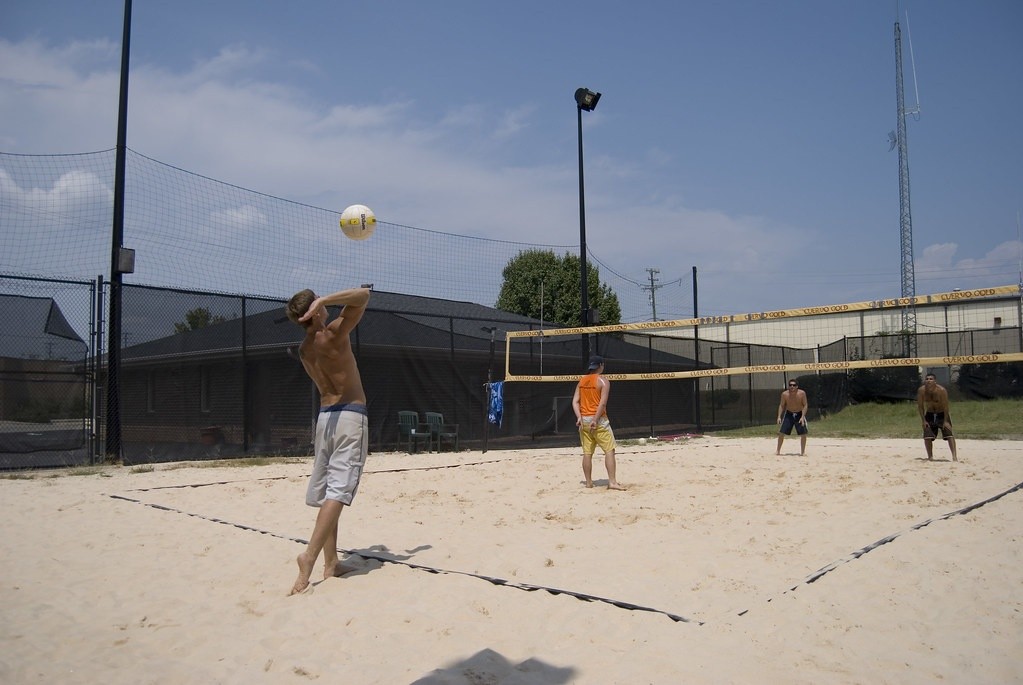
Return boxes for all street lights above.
[573,87,602,373]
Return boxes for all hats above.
[588,355,603,369]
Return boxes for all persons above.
[776,378,808,456]
[573,355,627,490]
[917,373,962,463]
[289,286,371,594]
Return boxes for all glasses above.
[925,377,933,381]
[789,384,797,386]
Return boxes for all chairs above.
[425,412,459,454]
[396,411,433,454]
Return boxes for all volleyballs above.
[339,204,377,239]
